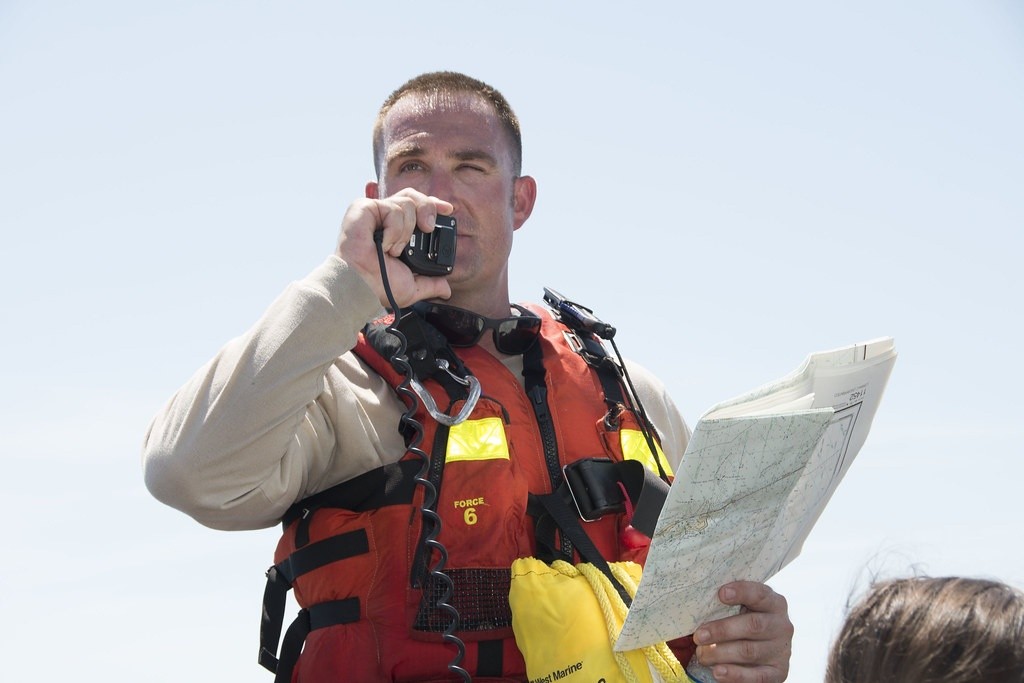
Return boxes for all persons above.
[142,71,795,683]
[824,575,1024,683]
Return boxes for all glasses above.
[412,300,542,356]
